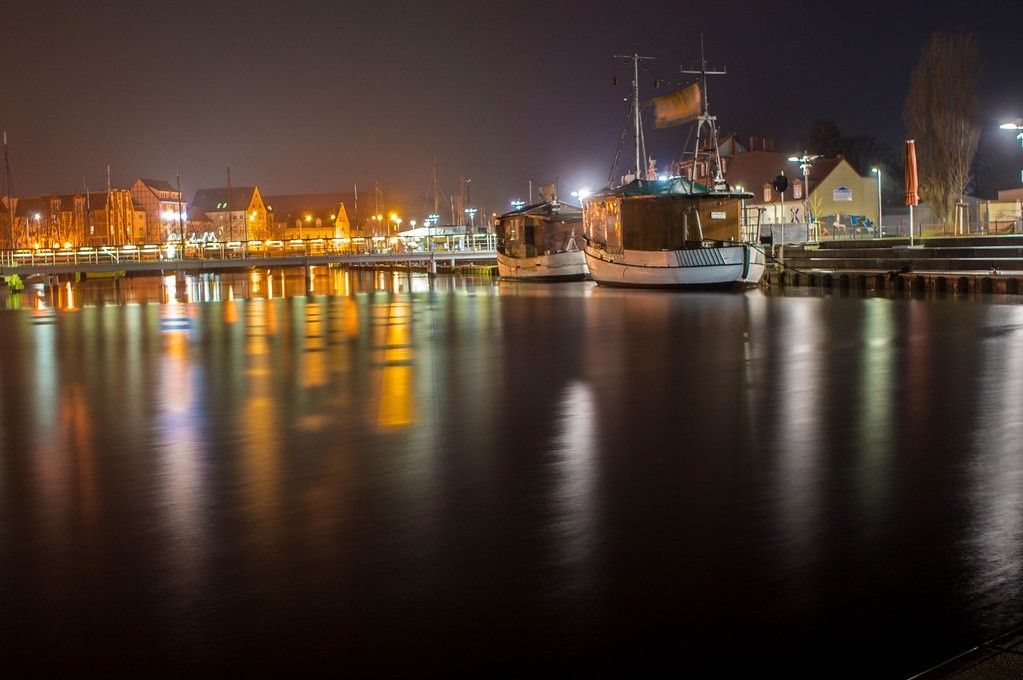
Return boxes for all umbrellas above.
[904,139,919,247]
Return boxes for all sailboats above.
[0,129,270,282]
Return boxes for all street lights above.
[409,220,416,230]
[465,209,477,233]
[511,200,525,211]
[396,219,402,232]
[429,215,439,235]
[789,155,825,222]
[306,216,311,238]
[425,219,436,234]
[572,191,588,210]
[872,166,883,238]
[331,215,335,238]
[424,222,430,228]
[378,216,382,236]
[387,216,395,236]
[34,213,41,244]
[372,216,376,234]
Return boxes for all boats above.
[579,23,768,289]
[497,199,590,282]
